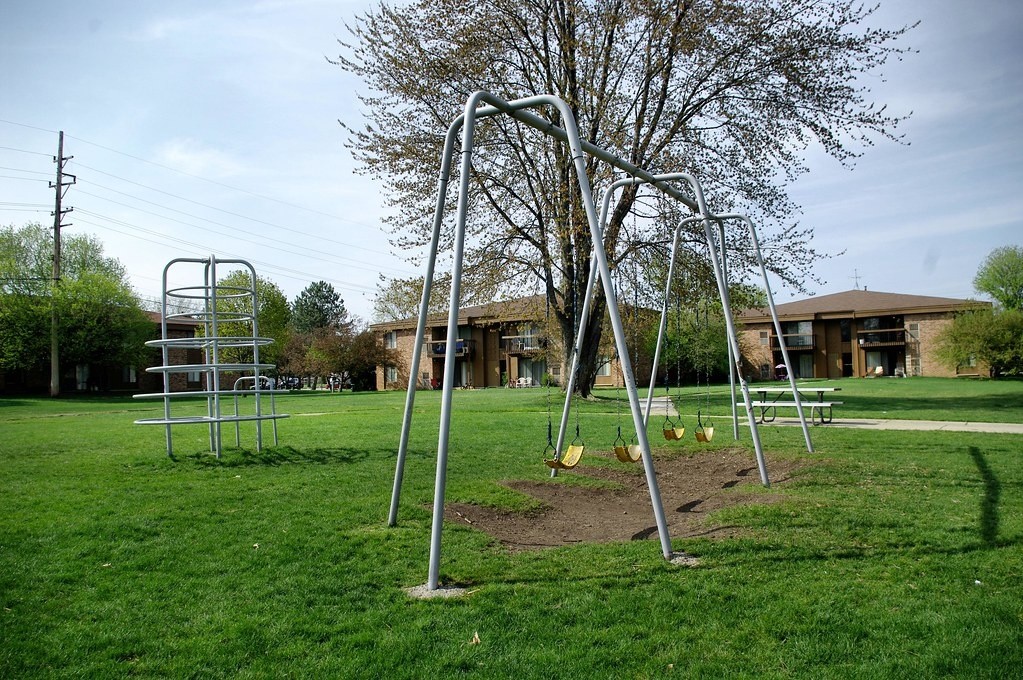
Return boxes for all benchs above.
[956,373,980,378]
[735,400,845,425]
[594,383,613,387]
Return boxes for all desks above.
[744,387,842,426]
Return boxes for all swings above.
[541,133,587,472]
[661,191,686,442]
[609,166,644,465]
[692,211,715,443]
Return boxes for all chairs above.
[515,376,533,389]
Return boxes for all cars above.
[280,378,304,389]
[326,381,352,390]
